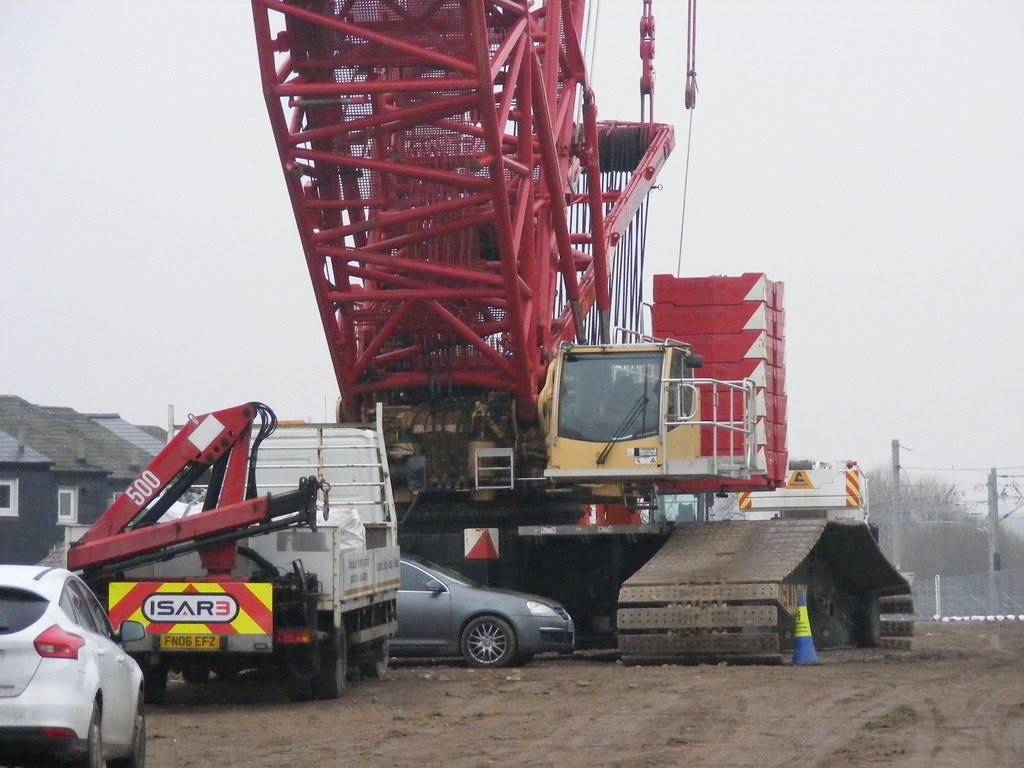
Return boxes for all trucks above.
[65,402,399,701]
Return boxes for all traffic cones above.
[789,590,819,667]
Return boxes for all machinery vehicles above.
[249,2,920,663]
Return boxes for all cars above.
[0,562,146,768]
[386,554,576,671]
